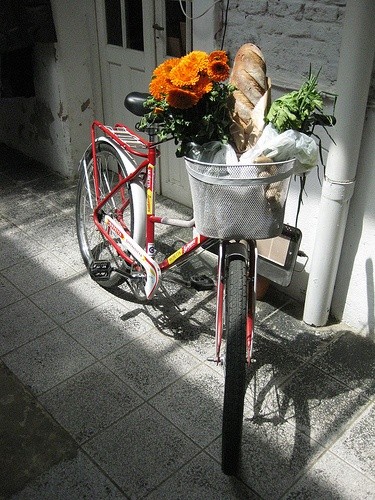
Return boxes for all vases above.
[185,138,226,165]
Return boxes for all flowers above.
[135,50,240,158]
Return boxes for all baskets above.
[184,142,295,239]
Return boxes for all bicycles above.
[71,92,336,477]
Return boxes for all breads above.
[254,158,281,210]
[226,43,271,153]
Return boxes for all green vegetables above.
[265,62,336,203]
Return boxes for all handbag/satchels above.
[187,122,319,239]
